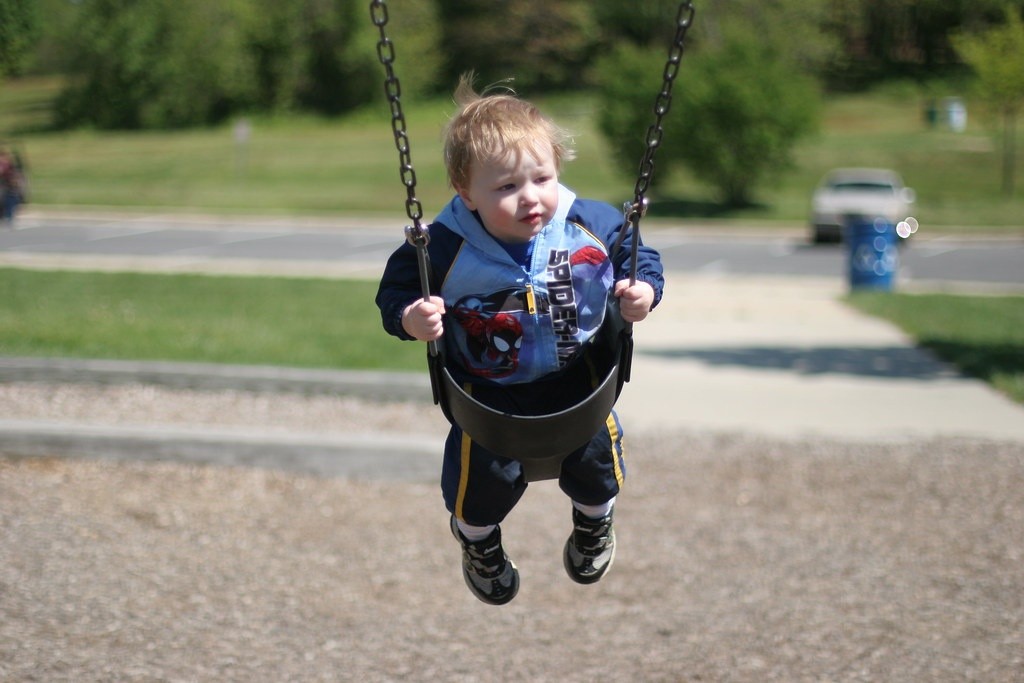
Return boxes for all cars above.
[809,167,916,245]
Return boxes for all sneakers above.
[450,514,520,605]
[562,504,616,584]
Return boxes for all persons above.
[375,96,665,604]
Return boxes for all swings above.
[366,0,701,487]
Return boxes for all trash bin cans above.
[849,220,899,291]
